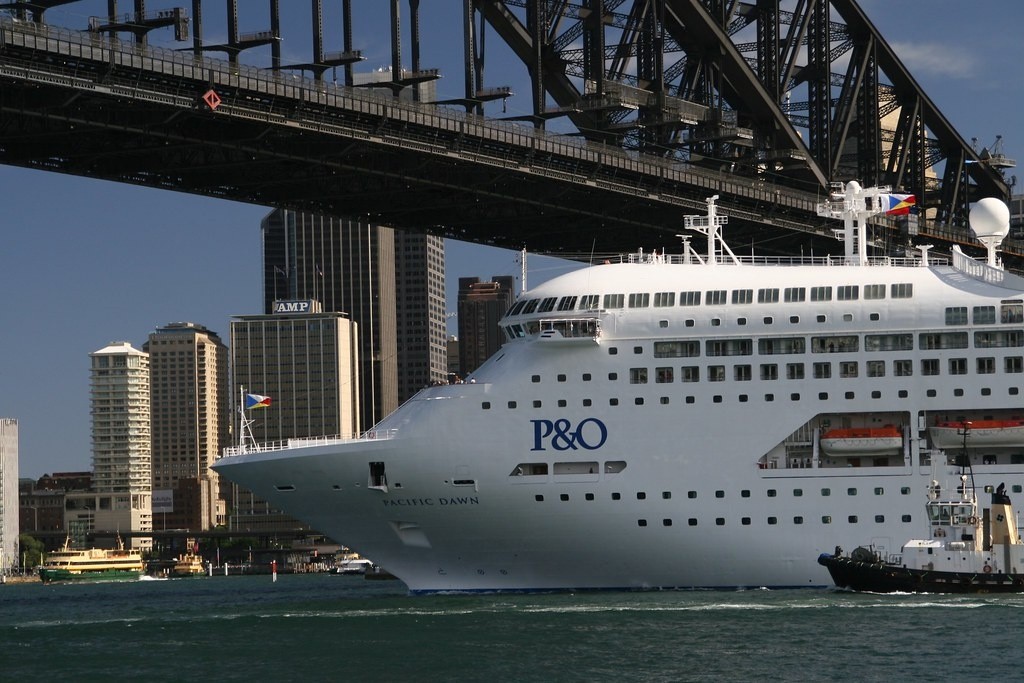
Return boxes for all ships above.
[210,179,1022,597]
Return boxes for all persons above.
[372,563,375,571]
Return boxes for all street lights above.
[161,506,165,531]
[31,507,38,531]
[85,505,91,531]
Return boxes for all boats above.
[168,550,208,577]
[819,424,903,457]
[38,530,148,585]
[927,417,1023,450]
[815,419,1023,594]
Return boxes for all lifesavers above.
[960,576,973,589]
[983,564,991,573]
[856,553,864,561]
[968,516,977,524]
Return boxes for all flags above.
[247,394,271,410]
[880,193,918,214]
